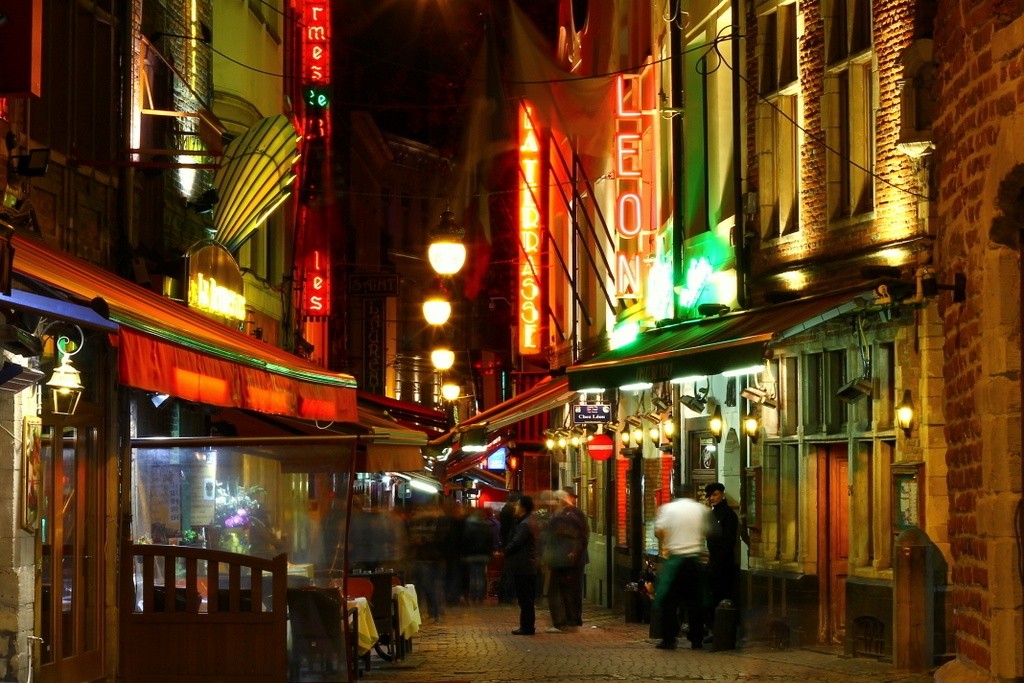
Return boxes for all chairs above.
[153,570,411,683]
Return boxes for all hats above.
[563,487,578,498]
[705,483,725,498]
[555,490,574,507]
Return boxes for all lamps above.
[862,264,901,279]
[37,319,85,415]
[698,303,731,317]
[895,389,913,437]
[836,314,873,404]
[921,272,966,302]
[603,401,619,432]
[744,405,760,443]
[681,376,710,415]
[741,357,777,408]
[183,189,217,214]
[9,147,53,175]
[428,202,467,277]
[543,422,598,455]
[620,381,674,448]
[764,291,800,302]
[707,404,723,442]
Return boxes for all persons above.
[324,482,738,654]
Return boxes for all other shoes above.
[545,626,579,633]
[655,639,677,649]
[704,635,714,645]
[691,641,703,648]
[513,627,535,634]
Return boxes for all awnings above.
[456,294,873,433]
[0,226,449,445]
